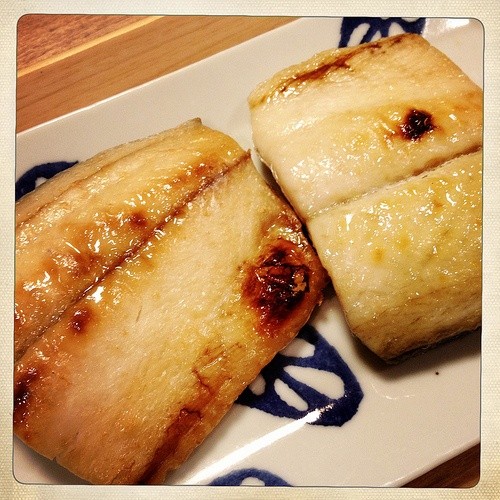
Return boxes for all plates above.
[15,16,484,487]
